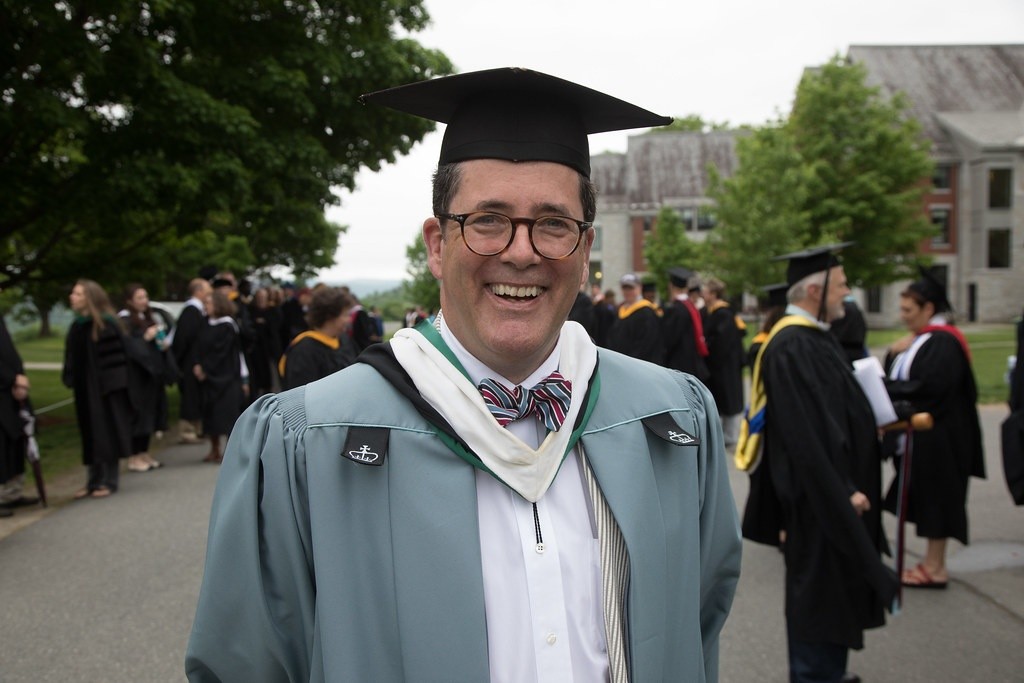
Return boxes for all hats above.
[766,241,860,322]
[358,65,674,162]
[620,274,640,287]
[642,278,658,291]
[667,268,690,287]
[912,263,951,314]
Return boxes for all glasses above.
[435,210,594,262]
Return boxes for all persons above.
[182,67,747,683]
[567,267,751,447]
[742,242,987,683]
[0,311,42,517]
[403,305,428,328]
[62,279,127,496]
[116,270,383,473]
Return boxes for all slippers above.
[903,561,950,592]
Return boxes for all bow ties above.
[478,371,574,427]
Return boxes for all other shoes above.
[75,486,115,497]
[119,455,160,471]
[1,495,40,516]
[172,419,202,445]
